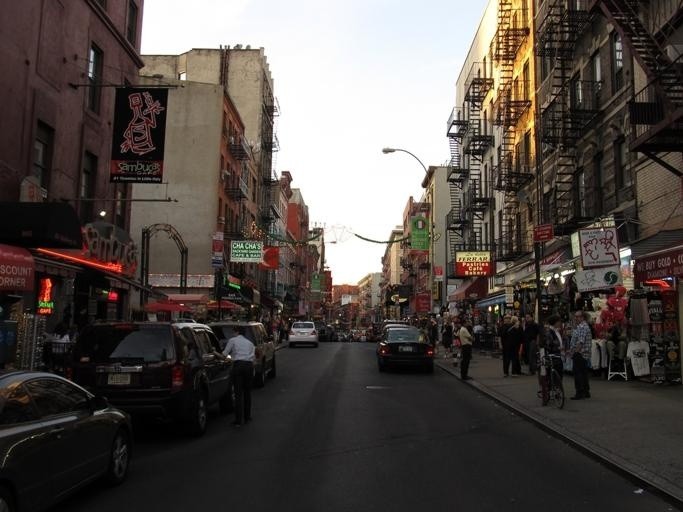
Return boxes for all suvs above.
[206,320,277,389]
[64,319,237,438]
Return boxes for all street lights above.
[317,241,336,249]
[382,147,435,316]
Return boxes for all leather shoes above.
[570,393,590,400]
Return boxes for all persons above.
[567,310,590,400]
[216,324,255,427]
[421,306,539,380]
[606,322,627,359]
[543,314,563,380]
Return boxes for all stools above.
[606,354,629,383]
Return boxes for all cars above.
[374,319,435,374]
[288,320,318,347]
[0,364,133,512]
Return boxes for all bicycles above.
[536,352,564,410]
